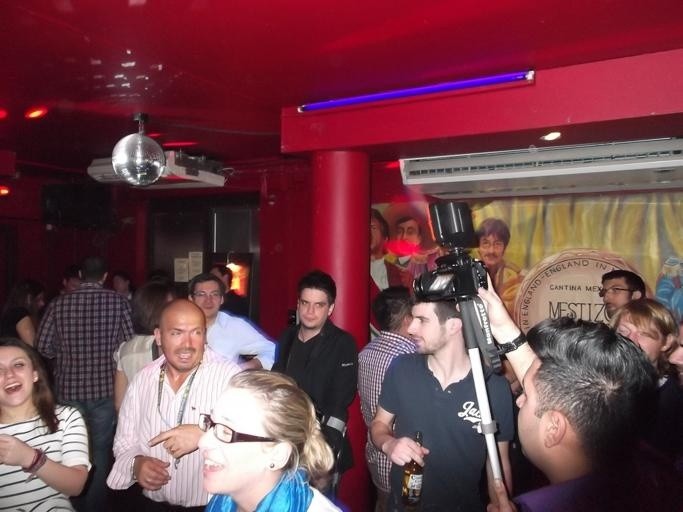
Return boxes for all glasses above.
[598,285,643,298]
[198,410,288,445]
[191,290,223,298]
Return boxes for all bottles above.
[400,431,425,503]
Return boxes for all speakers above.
[42,183,112,229]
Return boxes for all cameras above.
[412,201,488,303]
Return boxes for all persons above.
[386,214,425,289]
[472,218,527,313]
[1,252,357,512]
[366,209,409,332]
[358,269,683,512]
